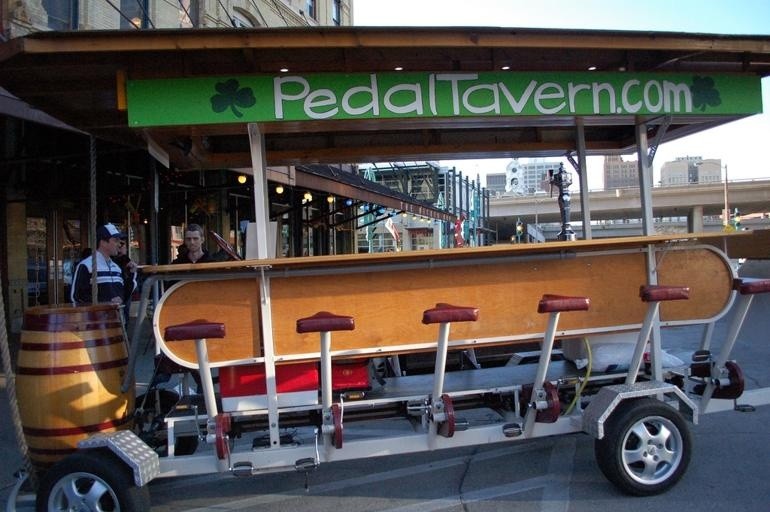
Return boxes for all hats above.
[97,224,127,240]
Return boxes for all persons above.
[71,221,137,330]
[169,224,213,263]
